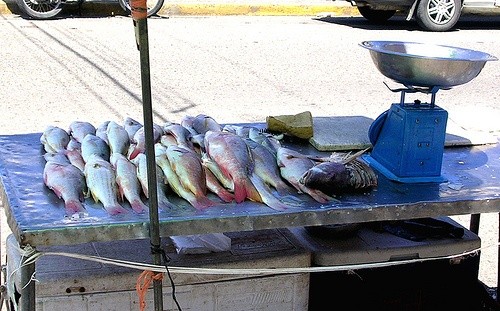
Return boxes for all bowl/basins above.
[358,41,498,87]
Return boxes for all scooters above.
[14,0,164,20]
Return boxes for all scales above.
[358,40,499,184]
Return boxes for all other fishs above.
[40,114,380,226]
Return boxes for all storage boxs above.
[286,216,480,311]
[6,228,309,311]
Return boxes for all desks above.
[0,122,500,311]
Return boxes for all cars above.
[328,0,500,32]
